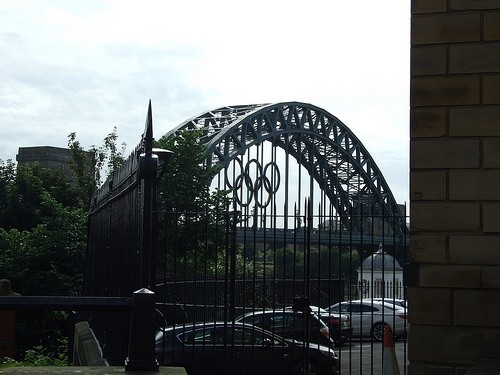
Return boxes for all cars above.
[232,309,336,350]
[324,300,410,343]
[283,305,351,345]
[156,322,340,375]
